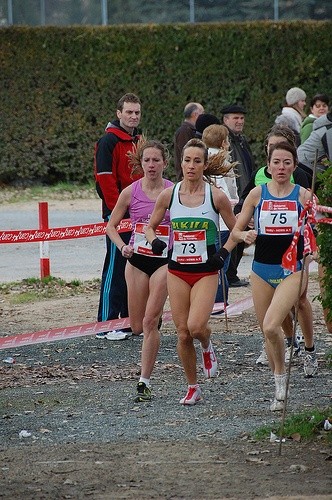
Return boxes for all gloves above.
[152,238,168,257]
[206,248,230,270]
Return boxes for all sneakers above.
[133,381,152,404]
[124,331,144,336]
[303,344,318,377]
[179,384,200,405]
[96,331,127,340]
[274,373,290,401]
[295,322,304,342]
[200,340,218,378]
[285,344,301,359]
[270,398,284,411]
[256,347,269,366]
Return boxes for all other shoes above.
[229,281,249,286]
[210,311,243,318]
[242,252,249,255]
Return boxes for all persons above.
[93,93,145,341]
[194,114,219,140]
[174,102,204,181]
[234,125,318,378]
[297,112,332,335]
[232,143,314,409]
[283,126,320,362]
[106,140,175,399]
[203,125,239,314]
[274,87,306,138]
[300,95,329,143]
[146,138,239,405]
[221,103,254,286]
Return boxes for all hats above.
[221,105,247,114]
[196,114,220,134]
[286,87,307,106]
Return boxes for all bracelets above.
[121,244,125,252]
